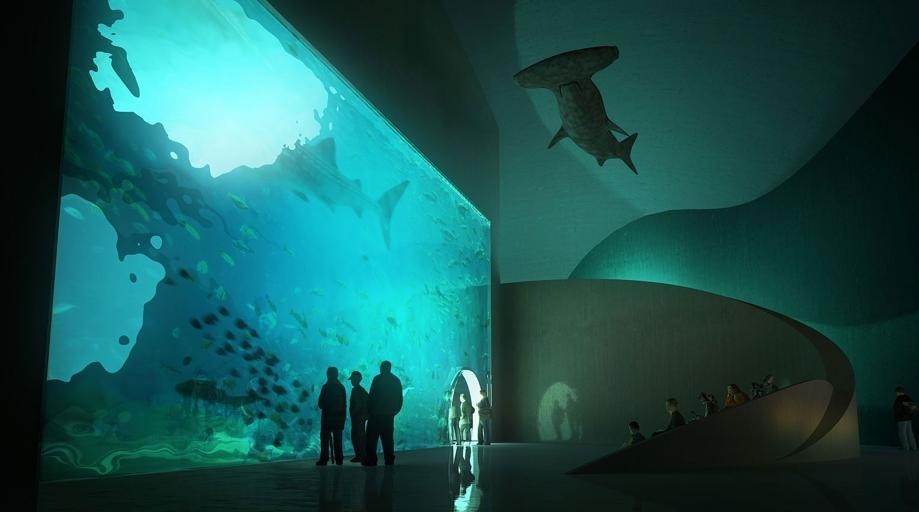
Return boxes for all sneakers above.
[316,455,394,466]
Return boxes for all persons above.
[893,384,917,452]
[650,398,686,437]
[621,420,644,448]
[346,370,371,463]
[450,391,462,446]
[689,374,777,420]
[314,366,348,467]
[460,392,475,444]
[476,390,493,446]
[365,359,403,466]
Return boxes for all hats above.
[348,371,361,380]
